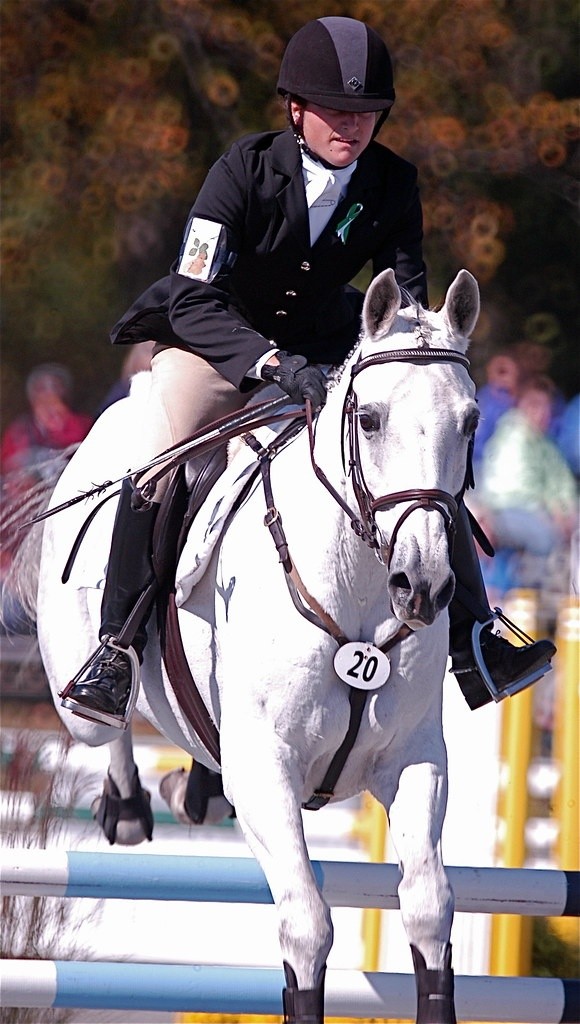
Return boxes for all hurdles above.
[0,848,579,1024]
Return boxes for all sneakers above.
[453,637,556,712]
[72,646,145,717]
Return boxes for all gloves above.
[262,349,329,408]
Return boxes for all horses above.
[3,266,478,1023]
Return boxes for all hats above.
[276,17,397,114]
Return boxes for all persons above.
[58,14,557,730]
[0,337,579,638]
